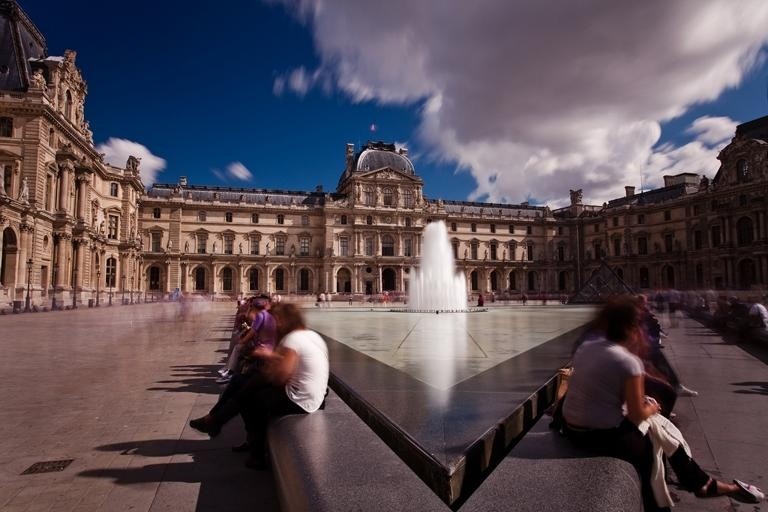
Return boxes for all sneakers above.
[189,417,221,437]
[733,478,764,502]
[233,442,261,454]
[215,368,233,384]
[677,386,698,398]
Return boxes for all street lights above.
[138,278,141,304]
[121,275,127,305]
[52,262,59,311]
[95,270,101,306]
[144,278,148,302]
[374,270,379,294]
[24,259,35,312]
[108,272,113,306]
[71,266,79,309]
[130,276,134,304]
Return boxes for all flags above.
[370,122,376,132]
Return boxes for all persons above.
[348,293,353,307]
[728,296,768,334]
[189,293,328,451]
[469,286,768,512]
[548,289,739,502]
[477,289,548,307]
